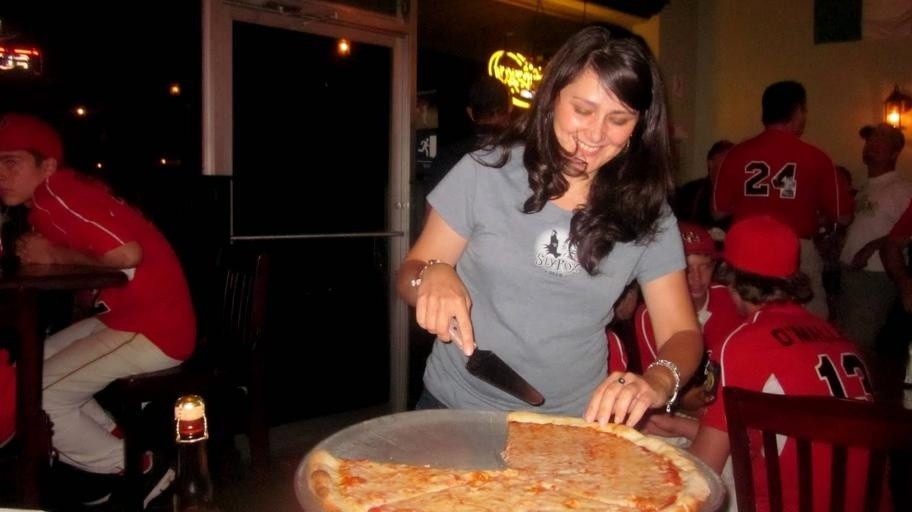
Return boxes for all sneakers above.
[40,471,112,505]
[140,448,178,511]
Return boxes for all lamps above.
[878,85,912,135]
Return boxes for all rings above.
[618,377,626,385]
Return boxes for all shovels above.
[448,317,545,405]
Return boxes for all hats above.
[859,122,905,151]
[723,211,803,279]
[0,115,63,162]
[675,223,719,259]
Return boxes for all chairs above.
[114,243,267,511]
[717,386,912,511]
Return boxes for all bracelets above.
[410,258,443,290]
[644,356,682,415]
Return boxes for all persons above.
[0,114,200,509]
[877,201,911,411]
[709,78,839,296]
[821,159,856,324]
[604,276,640,373]
[634,220,753,422]
[831,122,912,334]
[668,138,734,235]
[687,214,892,512]
[395,21,708,432]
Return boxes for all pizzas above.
[307,411,711,511]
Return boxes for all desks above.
[2,262,147,509]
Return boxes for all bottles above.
[172,393,214,511]
